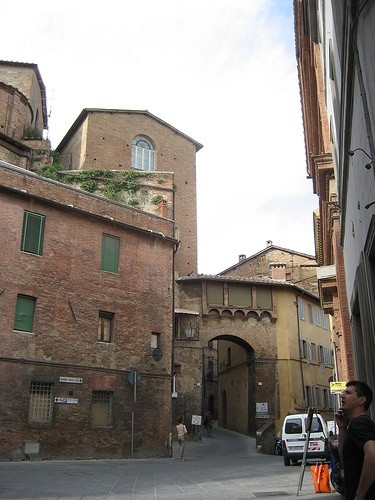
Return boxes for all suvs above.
[326,420,340,455]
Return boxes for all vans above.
[280,413,332,466]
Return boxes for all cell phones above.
[341,412,346,418]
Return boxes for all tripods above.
[296,407,334,496]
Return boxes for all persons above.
[335,381,375,500]
[204,411,214,437]
[176,419,188,461]
